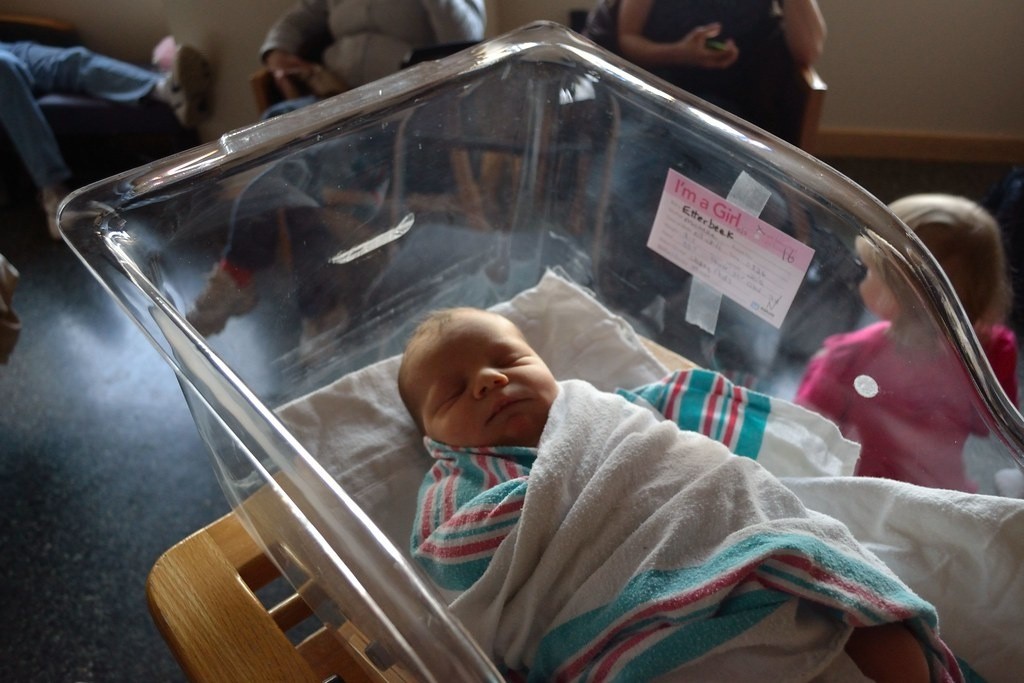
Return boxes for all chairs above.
[251,66,489,286]
[582,0,835,251]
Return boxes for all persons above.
[608,0,828,375]
[398,308,1024,683]
[0,42,204,240]
[185,0,490,360]
[798,192,1024,493]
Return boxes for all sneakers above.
[184,260,262,339]
[44,184,116,240]
[165,44,216,129]
